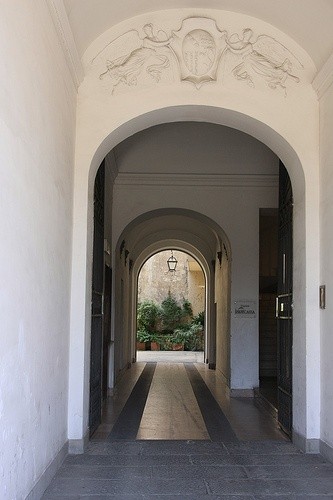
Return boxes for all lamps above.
[166,250,177,272]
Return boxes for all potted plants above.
[164,334,184,351]
[136,331,149,350]
[148,333,163,350]
